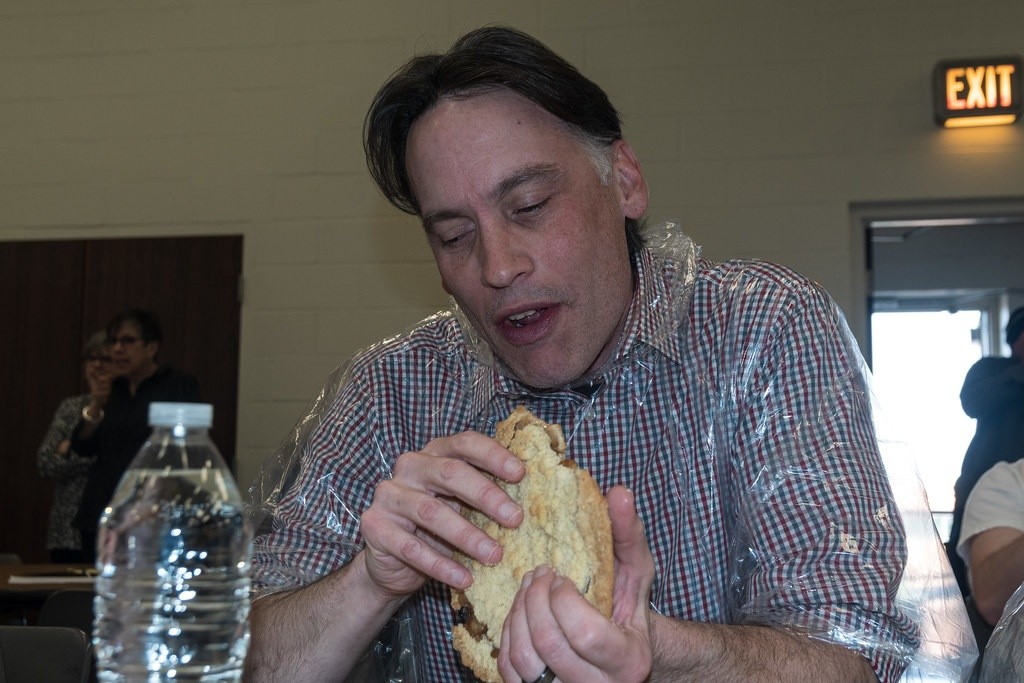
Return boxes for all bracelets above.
[82,405,105,422]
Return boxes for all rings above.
[521,664,556,683]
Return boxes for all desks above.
[1,553,95,592]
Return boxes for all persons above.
[72,311,216,569]
[946,306,1023,588]
[242,25,925,683]
[956,457,1024,620]
[34,327,123,560]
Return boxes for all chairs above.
[0,625,95,683]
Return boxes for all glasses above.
[84,354,113,363]
[102,335,137,347]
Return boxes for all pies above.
[449,404,615,683]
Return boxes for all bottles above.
[93,403,251,683]
